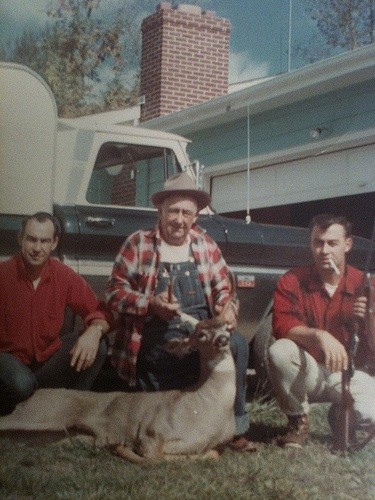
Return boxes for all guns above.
[332,229,375,450]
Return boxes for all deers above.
[0,268,237,465]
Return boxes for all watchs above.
[91,322,105,336]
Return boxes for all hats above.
[151,173,212,209]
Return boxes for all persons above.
[106,171,255,453]
[263,211,375,456]
[0,210,112,418]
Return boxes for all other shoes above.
[327,404,359,457]
[279,414,310,449]
[228,436,256,453]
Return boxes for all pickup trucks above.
[0,60,375,403]
